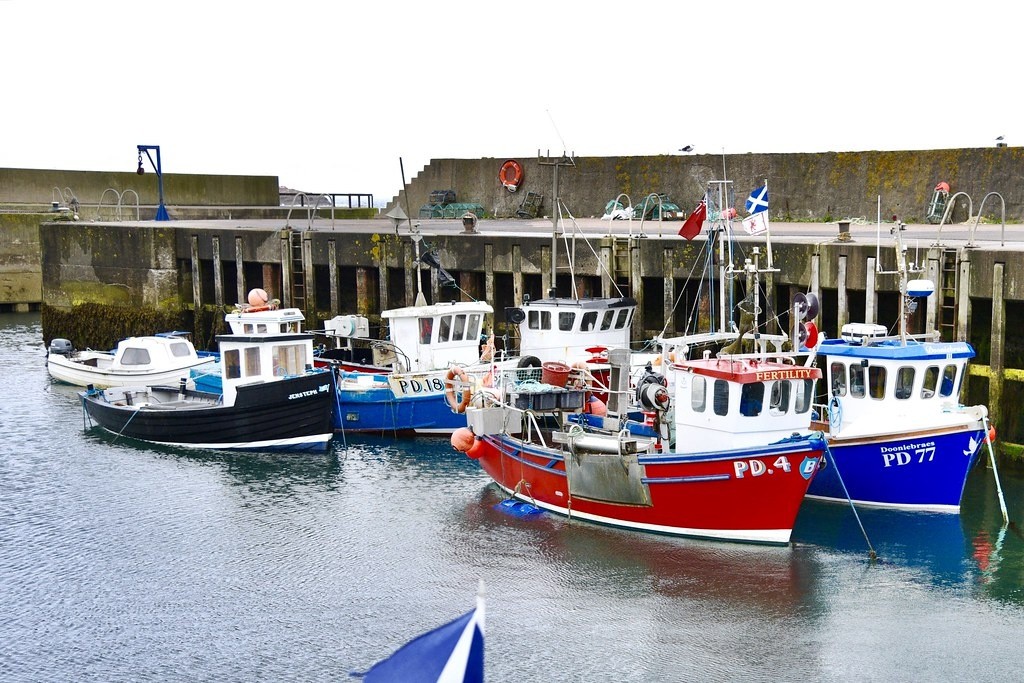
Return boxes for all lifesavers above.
[444,366,471,414]
[499,161,521,186]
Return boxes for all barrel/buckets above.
[541,361,571,389]
[586,359,610,406]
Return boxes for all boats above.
[460,148,828,548]
[316,158,638,408]
[111,310,524,433]
[569,196,992,515]
[77,288,342,453]
[47,335,214,390]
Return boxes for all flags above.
[678,202,706,242]
[745,185,769,215]
[742,210,770,235]
